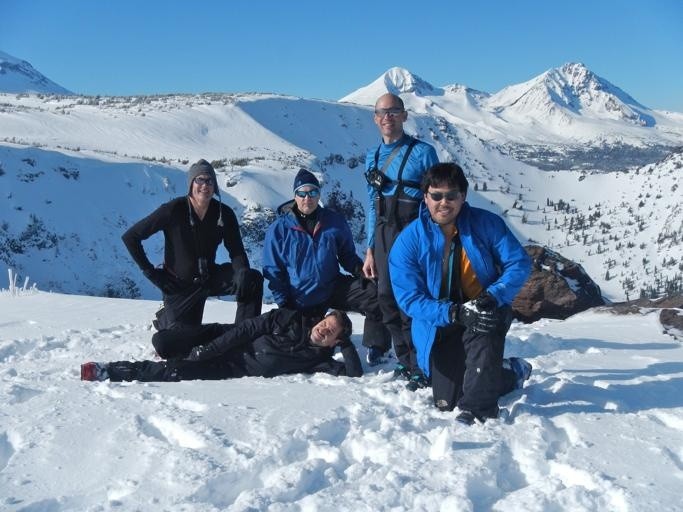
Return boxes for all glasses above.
[193,177,216,186]
[427,190,462,201]
[375,106,406,116]
[295,188,320,198]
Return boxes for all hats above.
[293,168,321,193]
[185,158,225,227]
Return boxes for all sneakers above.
[367,346,384,366]
[454,403,500,426]
[382,366,411,384]
[80,361,111,382]
[394,374,432,395]
[507,356,532,389]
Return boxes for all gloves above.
[450,294,499,335]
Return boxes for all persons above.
[263,169,398,366]
[81,309,364,382]
[121,158,263,359]
[362,94,440,391]
[388,163,532,424]
